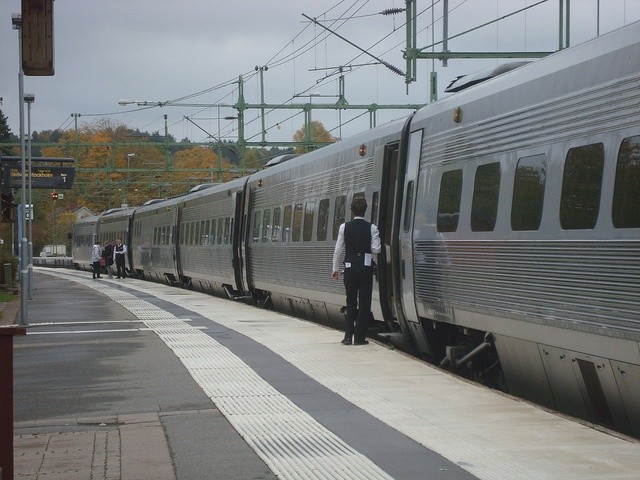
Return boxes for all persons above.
[91,241,103,279]
[113,238,128,279]
[333,198,381,346]
[103,242,116,279]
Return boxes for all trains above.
[72,21,639,440]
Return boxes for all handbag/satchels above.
[99,259,106,266]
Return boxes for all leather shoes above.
[354,341,368,344]
[341,338,352,344]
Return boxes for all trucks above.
[40,245,66,257]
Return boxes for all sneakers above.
[93,276,96,279]
[115,278,120,279]
[98,276,103,278]
